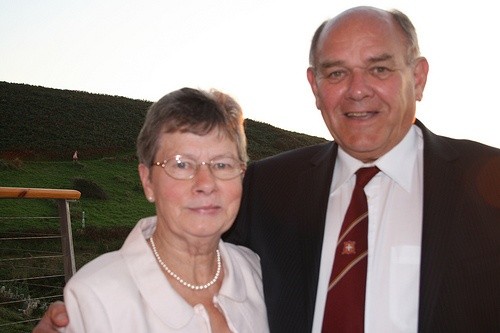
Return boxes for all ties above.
[321,165,381,333]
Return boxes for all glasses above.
[151,156,245,179]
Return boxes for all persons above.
[34,6,499,333]
[57,89,269,333]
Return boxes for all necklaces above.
[150,239,221,289]
